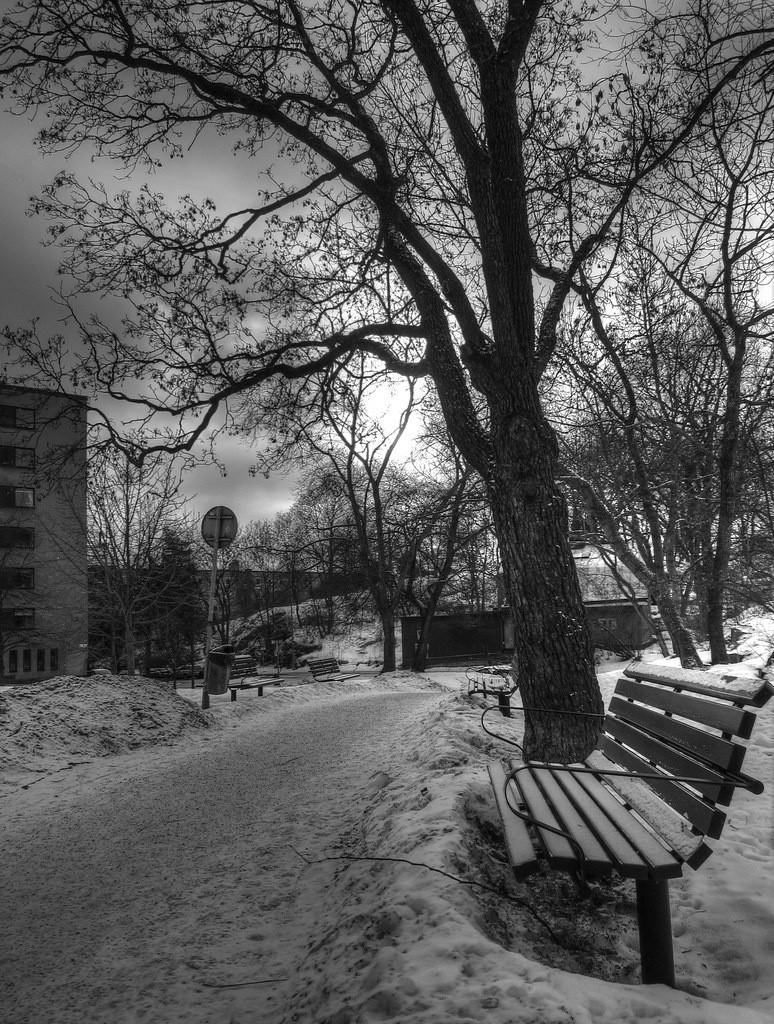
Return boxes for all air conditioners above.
[15,488,33,507]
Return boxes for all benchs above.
[307,657,360,683]
[467,655,520,718]
[481,661,774,990]
[228,655,285,702]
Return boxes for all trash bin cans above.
[207,643,236,696]
